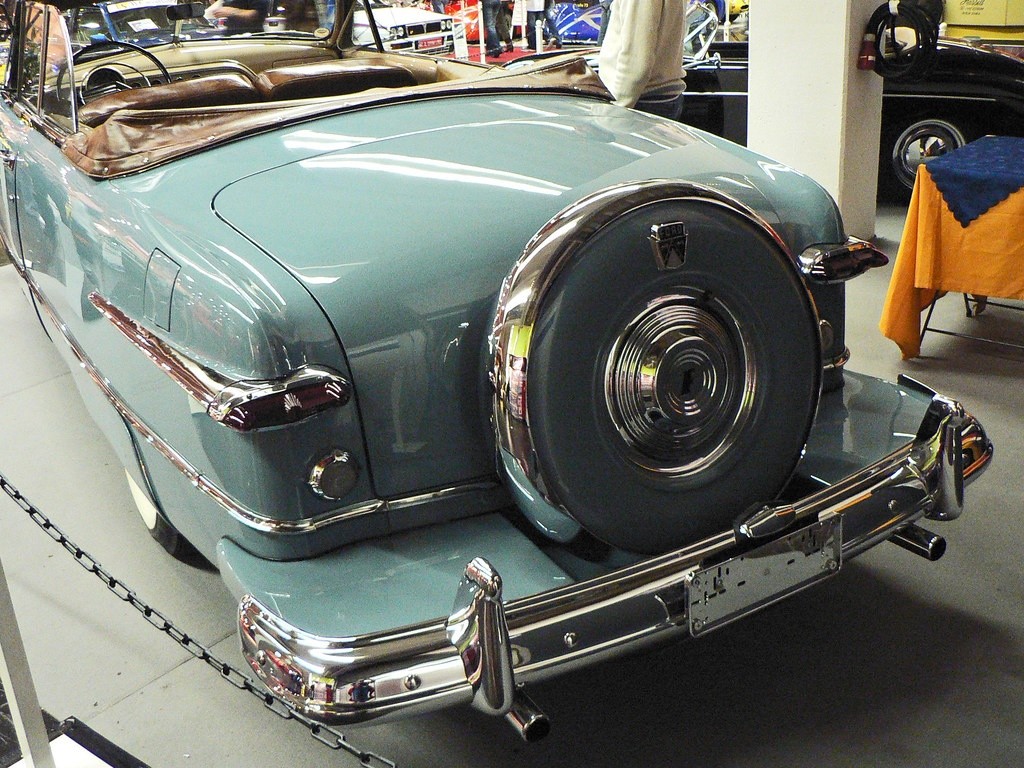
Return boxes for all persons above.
[496,0,515,53]
[594,0,689,122]
[211,0,268,32]
[525,0,545,49]
[481,0,504,57]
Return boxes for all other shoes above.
[503,45,514,52]
[484,45,503,58]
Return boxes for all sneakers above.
[520,46,536,53]
[556,42,562,49]
[544,38,556,51]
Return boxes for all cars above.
[354,0,455,58]
[2,0,996,745]
[501,2,1023,204]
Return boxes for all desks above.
[916,135,1024,359]
